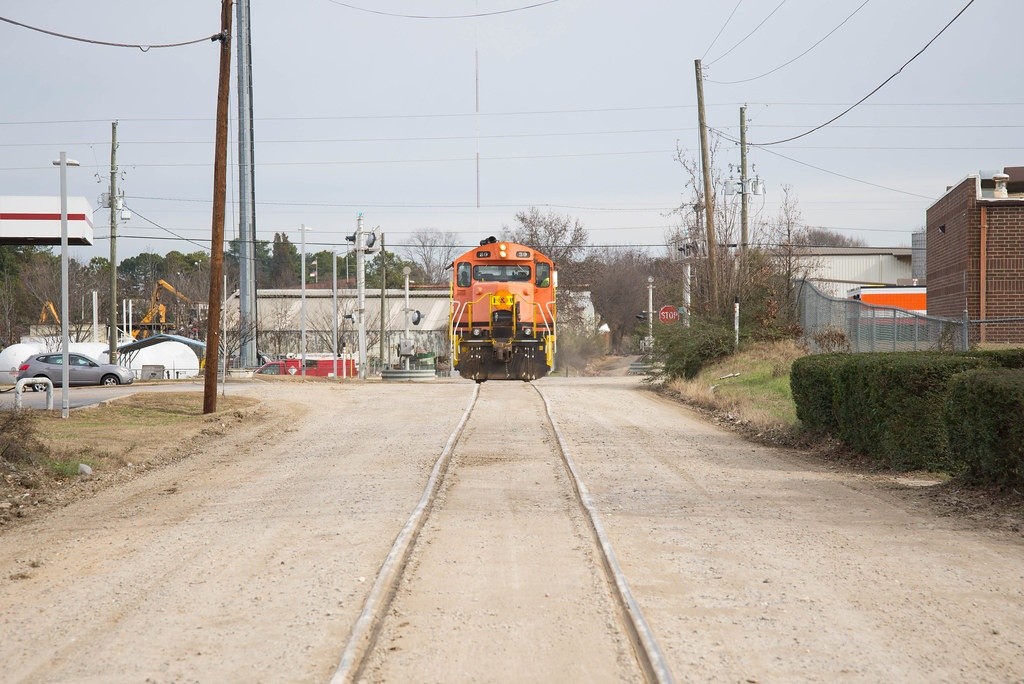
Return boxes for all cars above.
[16,353,135,392]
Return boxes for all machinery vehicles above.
[35,276,190,340]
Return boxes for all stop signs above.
[659,305,679,325]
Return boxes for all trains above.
[446,237,558,384]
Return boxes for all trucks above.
[253,358,359,378]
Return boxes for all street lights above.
[51,151,80,420]
